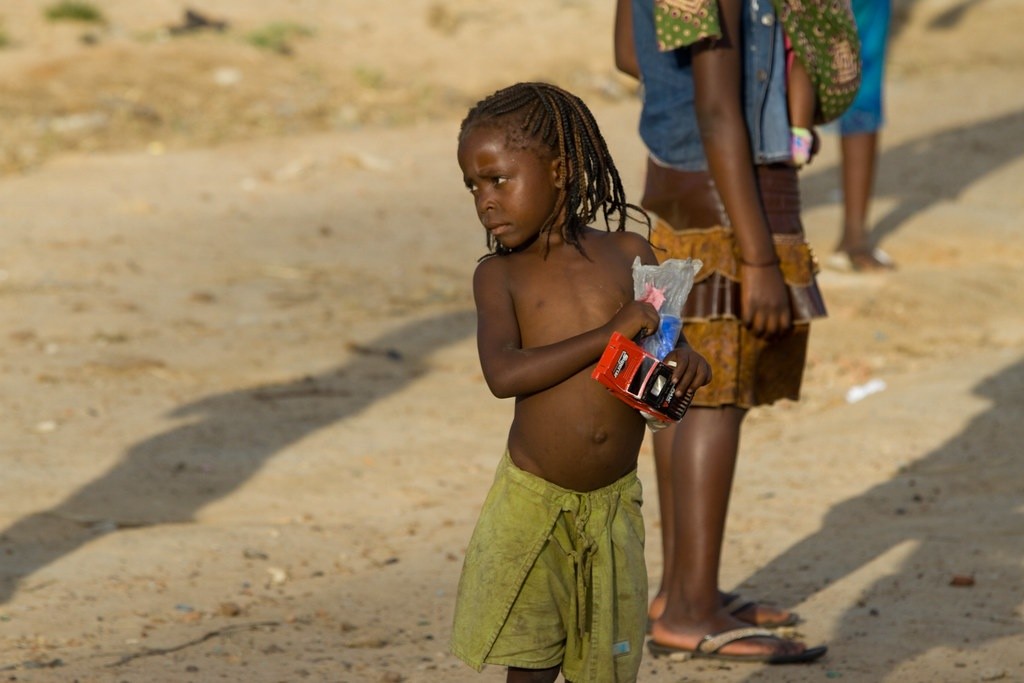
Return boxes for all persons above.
[614,0,898,665]
[450,82,713,683]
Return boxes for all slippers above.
[647,627,826,665]
[645,592,798,633]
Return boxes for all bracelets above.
[741,258,780,268]
[791,126,812,164]
